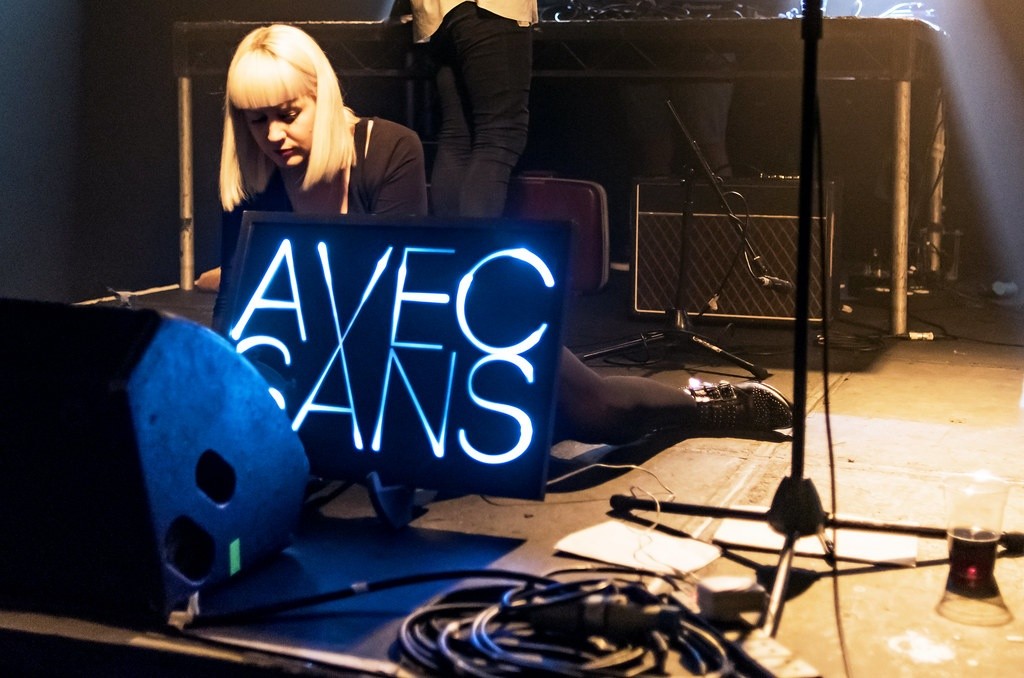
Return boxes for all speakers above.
[0,298,312,632]
[629,172,838,328]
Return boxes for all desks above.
[169,19,954,334]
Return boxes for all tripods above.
[608,0,1024,638]
[576,97,770,382]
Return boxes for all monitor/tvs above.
[215,211,578,501]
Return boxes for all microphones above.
[752,275,793,294]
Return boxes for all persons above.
[193,22,795,447]
[608,71,744,271]
[408,0,539,219]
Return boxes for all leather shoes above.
[678,380,794,434]
